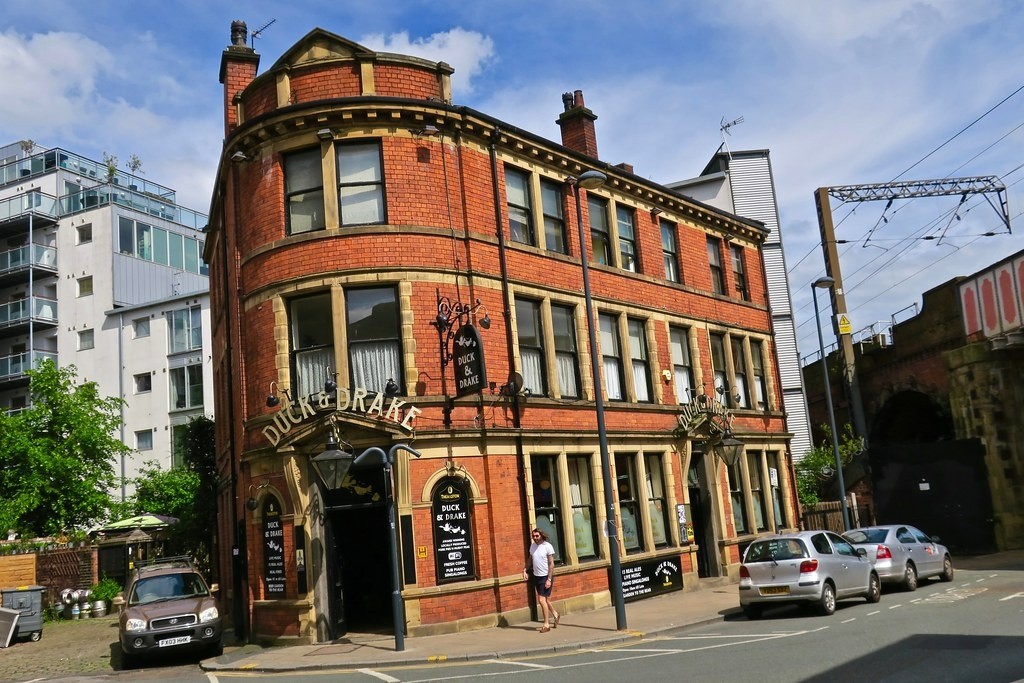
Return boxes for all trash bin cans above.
[0,585,47,642]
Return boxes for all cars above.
[119,554,224,665]
[739,531,882,615]
[840,525,954,590]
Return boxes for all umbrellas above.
[93,513,178,531]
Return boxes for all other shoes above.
[554,614,560,628]
[540,627,550,633]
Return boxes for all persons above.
[524,528,560,633]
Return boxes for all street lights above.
[563,171,629,631]
[810,276,850,534]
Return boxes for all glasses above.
[532,535,539,537]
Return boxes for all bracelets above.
[547,578,551,581]
